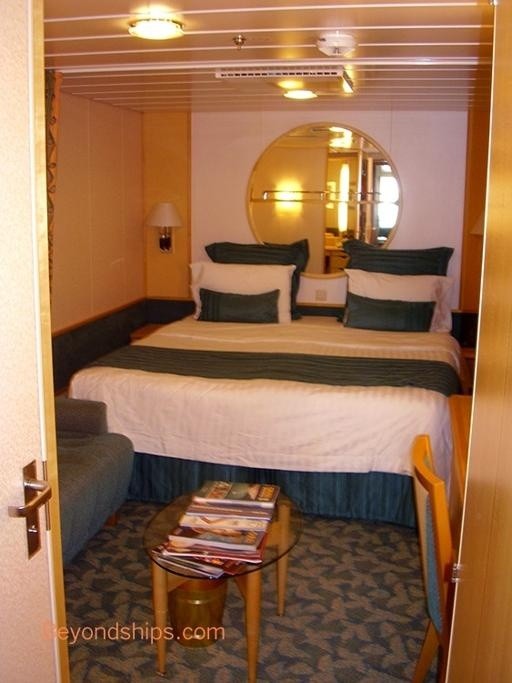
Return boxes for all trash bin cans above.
[167,579,228,647]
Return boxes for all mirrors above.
[245,120,403,279]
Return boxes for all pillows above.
[187,239,455,334]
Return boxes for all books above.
[151,479,280,579]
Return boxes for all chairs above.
[409,434,455,682]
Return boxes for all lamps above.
[145,202,183,254]
[128,18,187,40]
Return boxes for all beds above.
[68,303,457,523]
